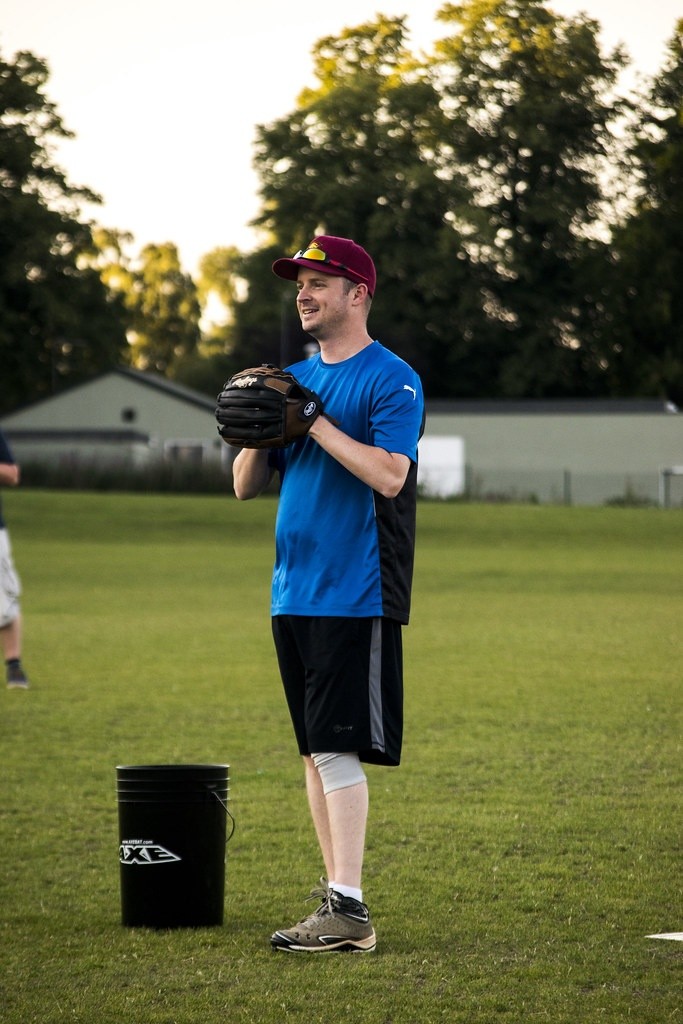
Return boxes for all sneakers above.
[270,877,377,955]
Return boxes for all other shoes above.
[4,668,28,689]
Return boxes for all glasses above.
[292,250,369,281]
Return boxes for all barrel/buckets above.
[117,765,235,929]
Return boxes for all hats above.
[273,236,377,299]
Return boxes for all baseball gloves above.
[214,362,326,451]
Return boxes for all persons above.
[216,237,426,955]
[0,436,29,689]
